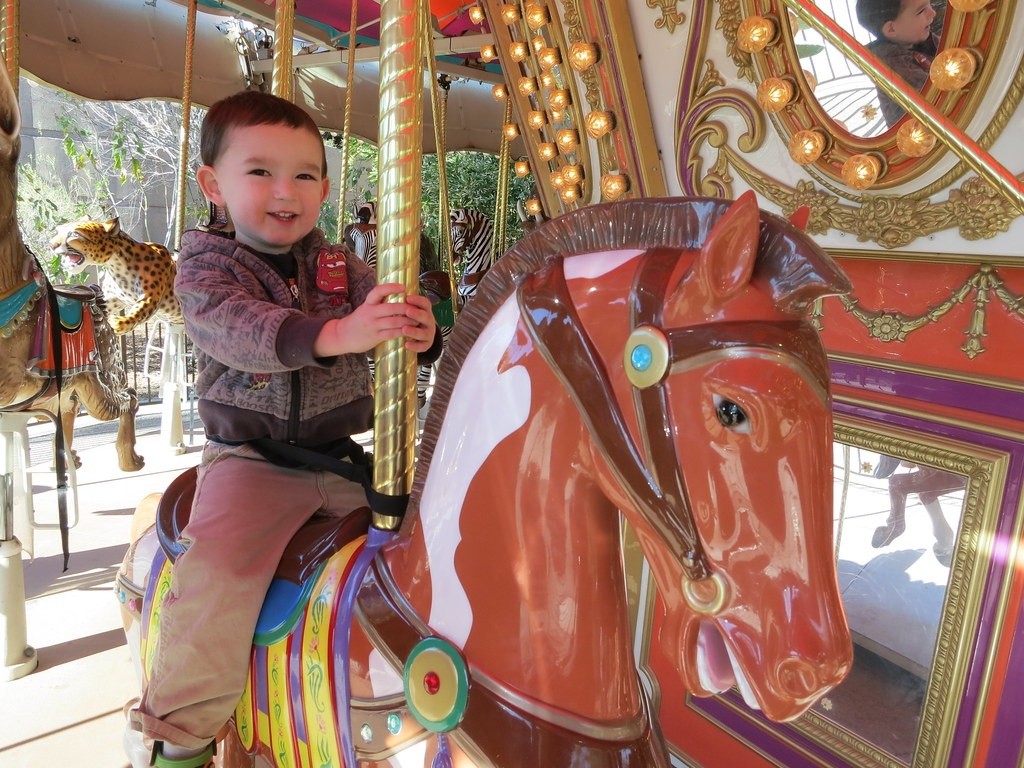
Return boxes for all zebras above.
[342,203,454,408]
[448,208,494,303]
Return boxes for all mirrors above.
[741,0,1013,193]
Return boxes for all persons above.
[852,0,942,131]
[126,89,446,768]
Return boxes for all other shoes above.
[150,738,217,768]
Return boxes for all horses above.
[0,57,147,472]
[115,192,854,768]
